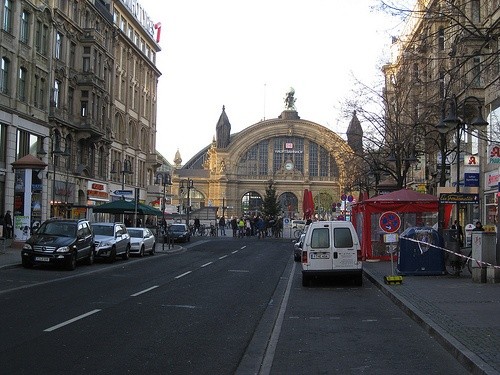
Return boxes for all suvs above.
[91,222,131,264]
[21,217,96,271]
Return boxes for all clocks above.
[285,161,295,171]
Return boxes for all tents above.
[350,187,441,263]
[92,199,163,227]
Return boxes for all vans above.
[299,221,363,287]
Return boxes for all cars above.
[292,233,306,262]
[126,228,156,257]
[164,224,190,243]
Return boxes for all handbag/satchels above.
[6,224,12,228]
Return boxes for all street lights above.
[110,159,134,224]
[179,177,194,225]
[154,172,172,225]
[434,94,489,278]
[37,135,71,219]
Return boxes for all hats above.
[7,210,12,212]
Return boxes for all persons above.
[472,221,485,231]
[231,216,283,240]
[194,216,200,232]
[162,219,167,234]
[452,220,464,247]
[219,217,226,236]
[3,210,13,239]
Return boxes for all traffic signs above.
[113,190,133,195]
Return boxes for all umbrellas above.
[303,188,314,220]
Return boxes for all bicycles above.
[444,242,473,276]
[293,228,304,239]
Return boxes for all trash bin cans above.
[397,227,446,275]
[443,229,460,277]
